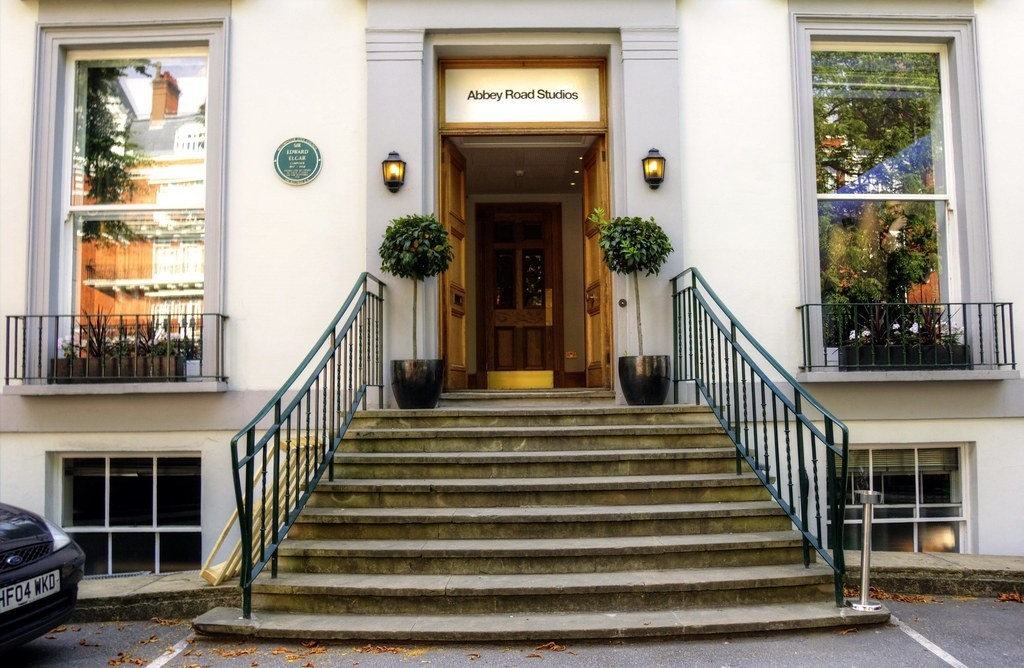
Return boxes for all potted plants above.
[586,205,676,404]
[51,303,189,382]
[377,211,455,409]
[837,278,972,371]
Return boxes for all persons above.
[879,200,930,303]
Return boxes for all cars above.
[0,502,86,657]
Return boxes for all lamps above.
[641,146,667,190]
[380,149,408,194]
